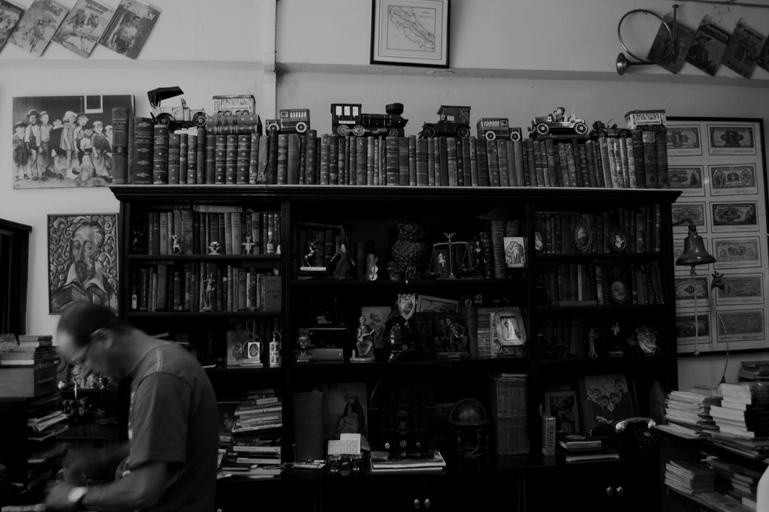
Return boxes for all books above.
[646,15,695,74]
[664,357,769,511]
[0,331,70,464]
[8,0,69,57]
[723,20,766,80]
[755,34,768,73]
[51,0,113,59]
[685,20,731,77]
[0,0,26,57]
[98,1,161,60]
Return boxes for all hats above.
[27,110,39,118]
[62,110,77,122]
[14,121,27,128]
[77,115,89,122]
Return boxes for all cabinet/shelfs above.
[110,181,683,512]
[1,330,109,511]
[654,358,768,511]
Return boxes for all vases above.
[369,0,451,68]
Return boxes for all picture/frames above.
[46,214,120,316]
[666,114,768,357]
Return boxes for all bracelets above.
[67,482,87,509]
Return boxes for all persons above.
[51,220,117,310]
[9,107,115,185]
[686,32,711,72]
[110,16,142,53]
[41,298,221,512]
